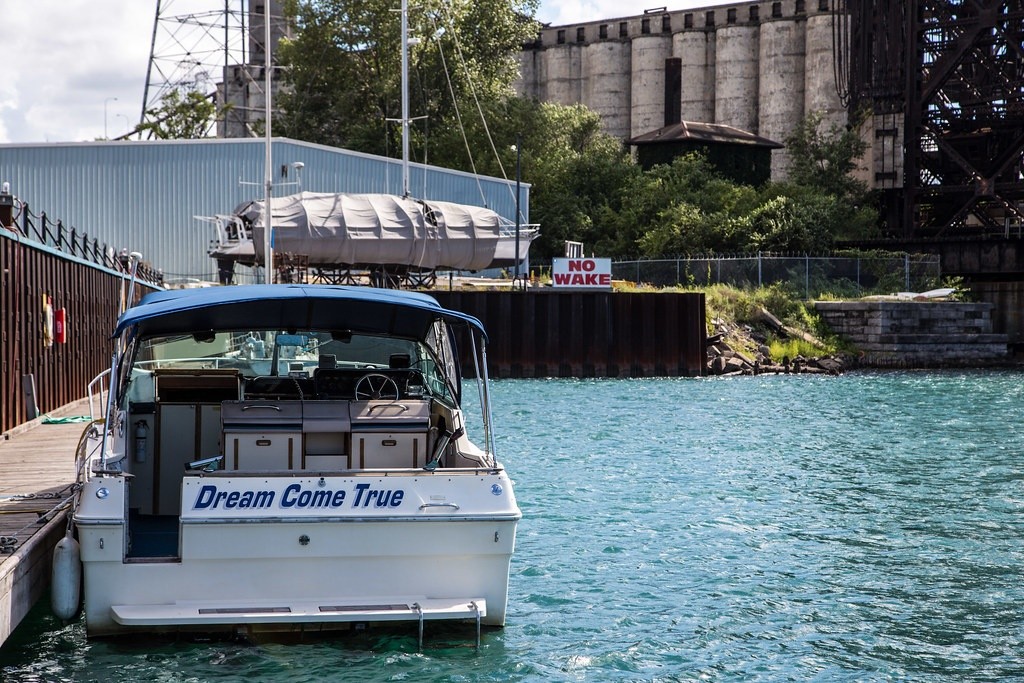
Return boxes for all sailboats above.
[54,0,541,642]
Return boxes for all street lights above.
[104,97,118,138]
[116,113,130,138]
